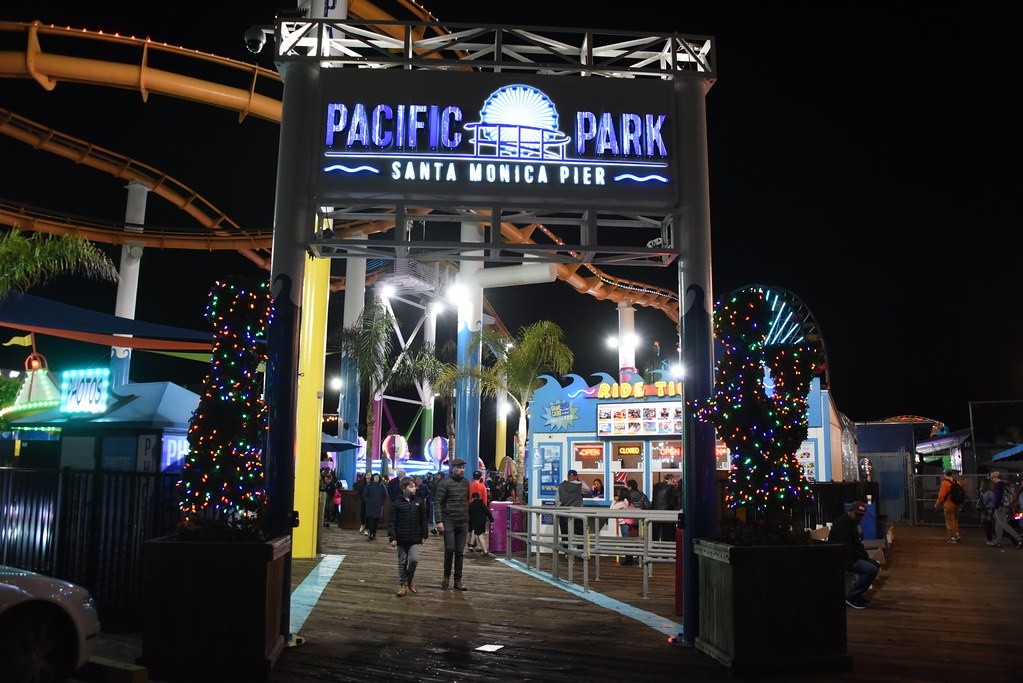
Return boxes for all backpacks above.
[623,501,635,525]
[975,491,991,511]
[999,481,1014,507]
[944,478,964,506]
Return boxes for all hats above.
[451,458,467,465]
[989,472,1000,479]
[943,468,952,473]
[568,470,577,476]
[850,501,866,516]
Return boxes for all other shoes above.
[474,541,478,547]
[468,544,475,552]
[358,524,365,532]
[364,530,368,536]
[987,540,994,545]
[368,534,376,540]
[475,548,482,552]
[484,551,489,556]
[1015,540,1023,549]
[991,541,1000,547]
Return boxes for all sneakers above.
[453,580,467,591]
[408,579,417,593]
[845,594,869,609]
[441,573,450,589]
[397,583,407,596]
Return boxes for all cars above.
[0,565,99,683]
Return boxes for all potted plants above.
[693,289,853,674]
[135,262,291,683]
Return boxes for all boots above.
[621,555,633,565]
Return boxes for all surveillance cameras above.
[244,28,266,53]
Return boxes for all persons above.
[387,470,445,502]
[359,472,388,540]
[652,474,683,542]
[487,475,516,502]
[827,500,880,609]
[610,488,634,566]
[433,459,470,591]
[979,471,1023,549]
[387,477,429,596]
[468,470,488,547]
[626,479,651,525]
[934,468,962,543]
[558,470,584,559]
[587,478,604,498]
[320,469,337,523]
[468,492,494,556]
